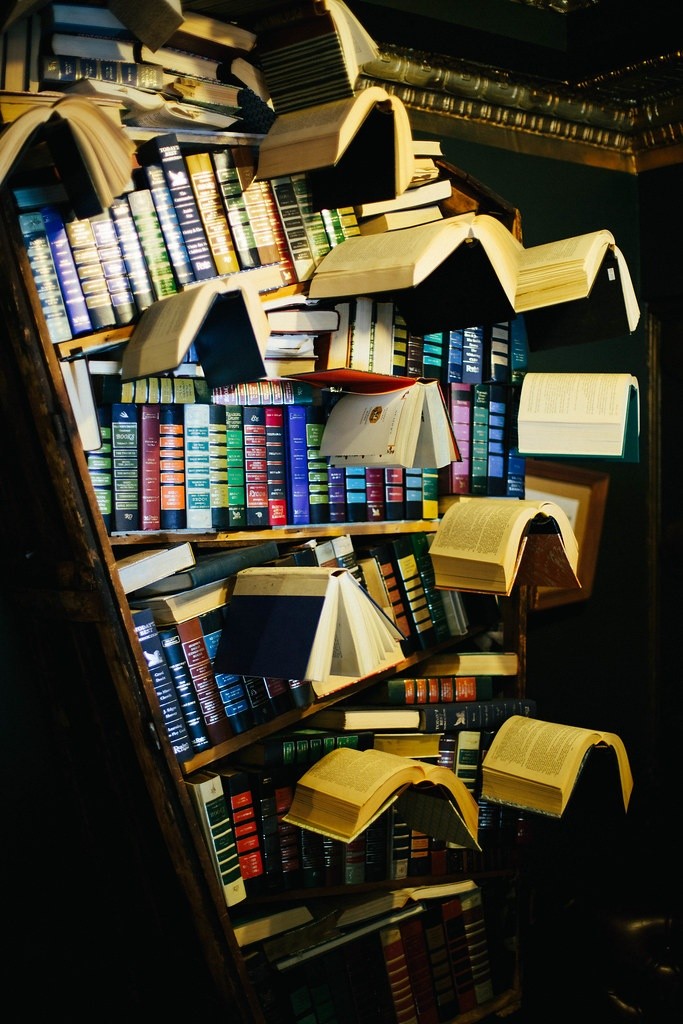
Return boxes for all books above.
[517,373,641,463]
[514,229,642,352]
[428,497,583,597]
[482,715,634,817]
[282,747,482,853]
[213,566,409,682]
[0,0,527,1023]
